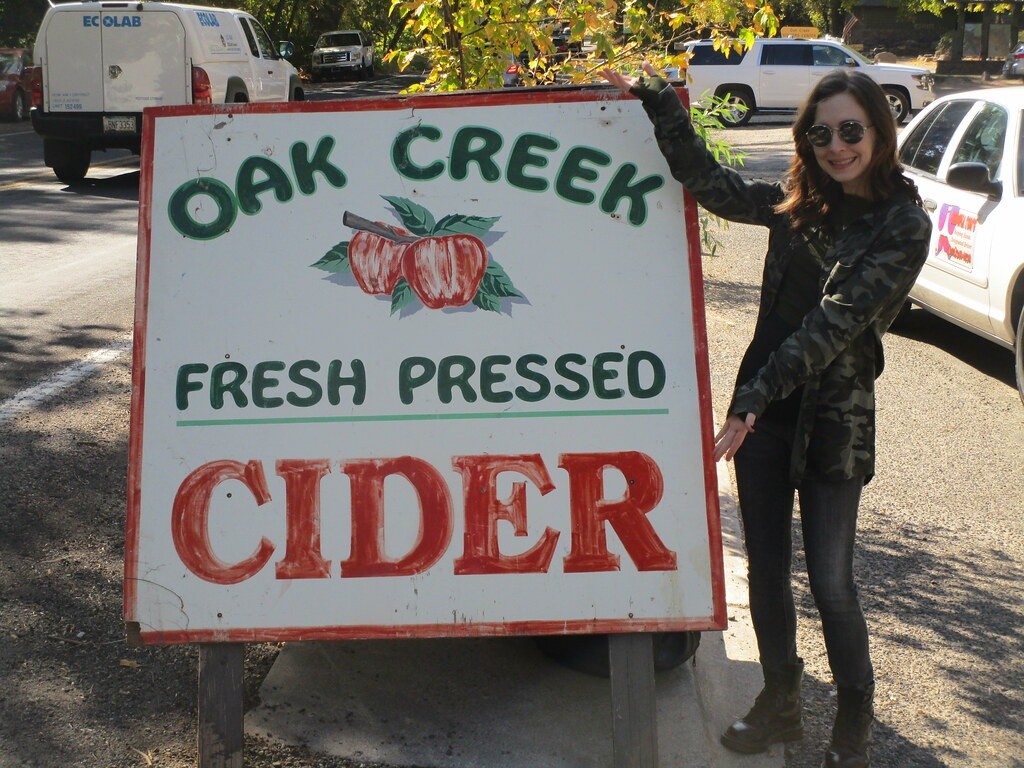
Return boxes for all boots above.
[819,680,874,768]
[720,658,804,753]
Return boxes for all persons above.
[598,61,933,768]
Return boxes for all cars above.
[1002,41,1024,76]
[0,48,33,123]
[308,29,375,82]
[895,86,1024,404]
[502,17,585,88]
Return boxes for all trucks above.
[29,1,305,185]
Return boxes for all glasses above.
[803,121,873,147]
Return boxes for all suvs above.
[682,37,936,129]
